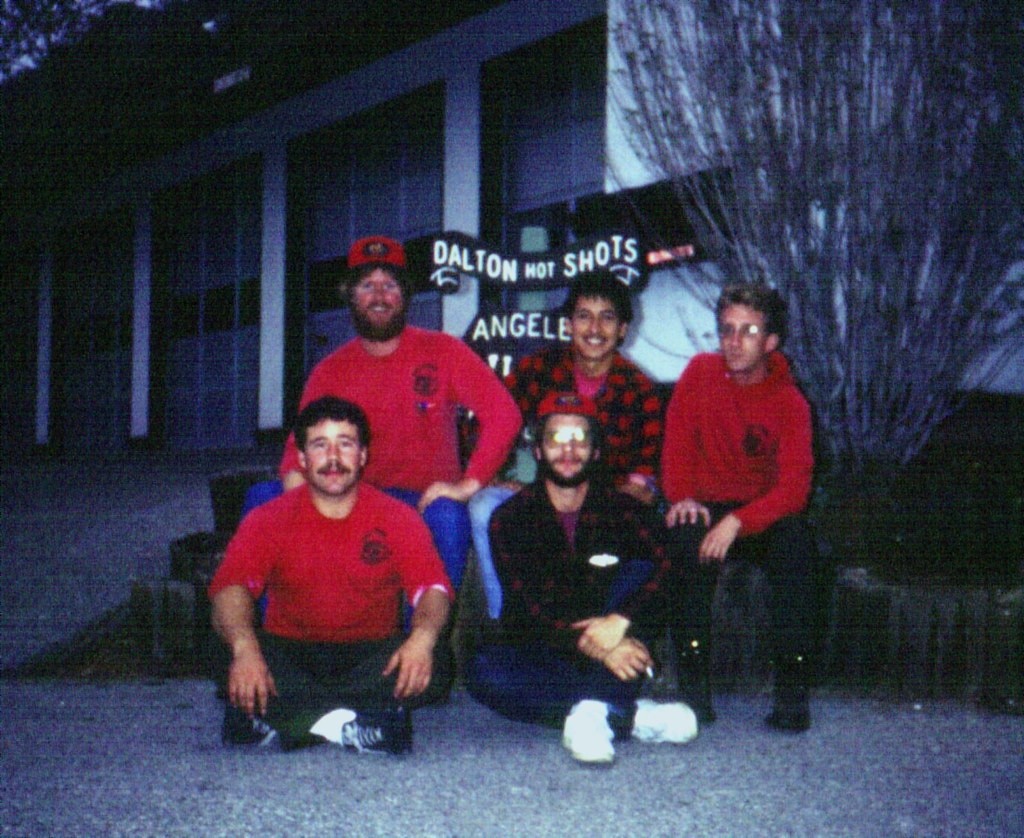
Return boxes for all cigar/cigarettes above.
[645,663,654,679]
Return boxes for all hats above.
[346,236,406,269]
[536,390,597,420]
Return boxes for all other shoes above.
[629,698,700,746]
[560,698,616,763]
[340,708,410,757]
[763,706,811,732]
[220,705,278,750]
[691,706,718,724]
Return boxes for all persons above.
[461,391,697,763]
[238,235,524,711]
[457,267,669,620]
[660,285,823,731]
[206,395,454,757]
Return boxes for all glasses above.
[305,438,360,455]
[358,281,398,293]
[717,326,764,336]
[542,430,592,448]
[572,310,618,323]
